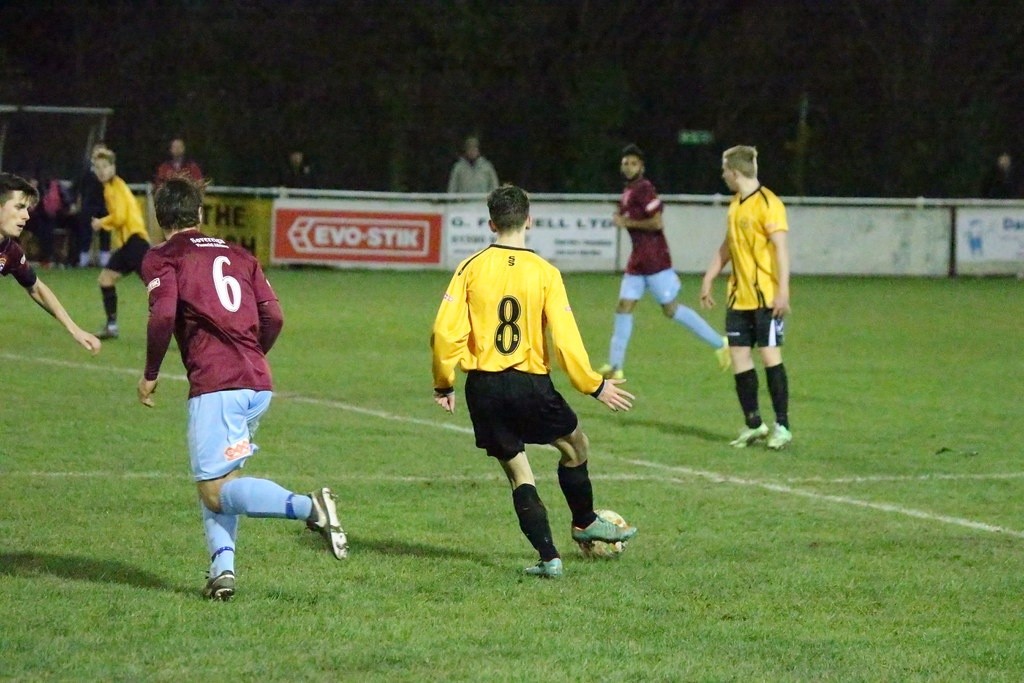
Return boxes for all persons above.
[0,172,102,358]
[90,147,154,338]
[281,149,310,188]
[25,174,92,266]
[595,143,730,380]
[430,184,638,578]
[700,144,795,452]
[155,140,205,196]
[137,178,348,600]
[449,137,499,192]
[980,152,1024,200]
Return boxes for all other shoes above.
[92,327,118,340]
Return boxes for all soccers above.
[579,509,629,559]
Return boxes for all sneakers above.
[202,570,236,602]
[571,514,637,543]
[715,336,731,371]
[524,558,562,576]
[304,487,348,560]
[767,424,793,450]
[729,423,769,448]
[598,364,625,380]
